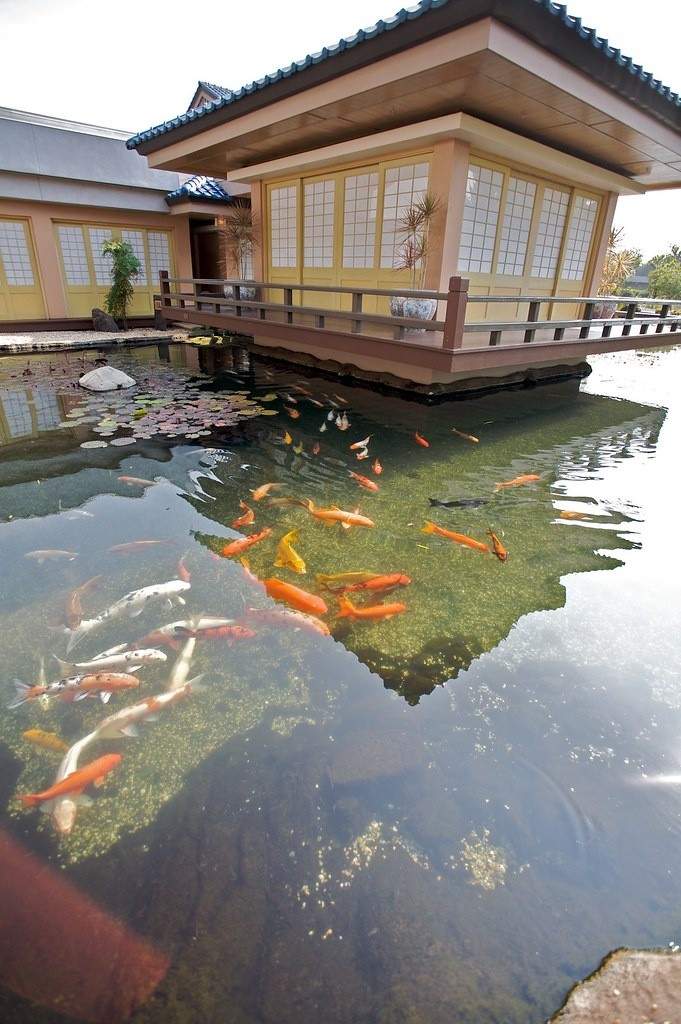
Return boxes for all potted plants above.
[592,226,643,319]
[390,191,448,333]
[218,197,261,311]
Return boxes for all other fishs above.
[8,637,206,835]
[261,368,585,529]
[23,448,507,635]
